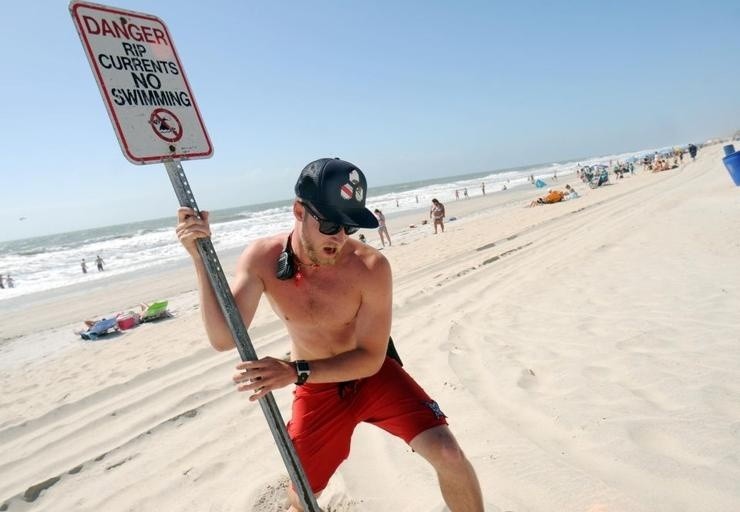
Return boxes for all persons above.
[613,142,698,180]
[374,209,392,246]
[176,158,483,512]
[5,275,15,288]
[0,274,6,288]
[95,255,105,271]
[479,182,486,194]
[430,198,444,234]
[80,259,86,273]
[565,185,578,199]
[463,188,470,199]
[454,189,460,201]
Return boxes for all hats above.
[295,157,380,230]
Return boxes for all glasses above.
[299,201,360,236]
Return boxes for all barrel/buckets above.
[722,150,740,187]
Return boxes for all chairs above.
[541,191,561,203]
[80,299,169,342]
[591,174,611,187]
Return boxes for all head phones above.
[276,228,296,281]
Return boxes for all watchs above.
[294,360,311,386]
